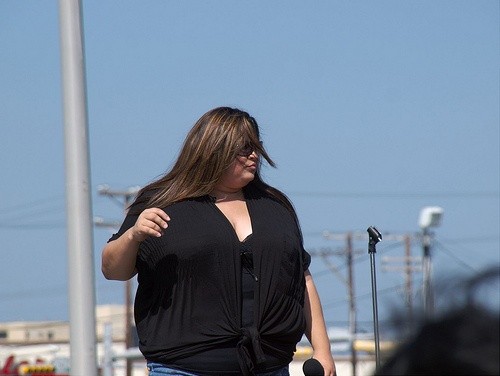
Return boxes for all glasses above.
[236,141,263,157]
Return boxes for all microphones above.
[302,359,324,376]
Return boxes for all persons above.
[101,107,336,376]
